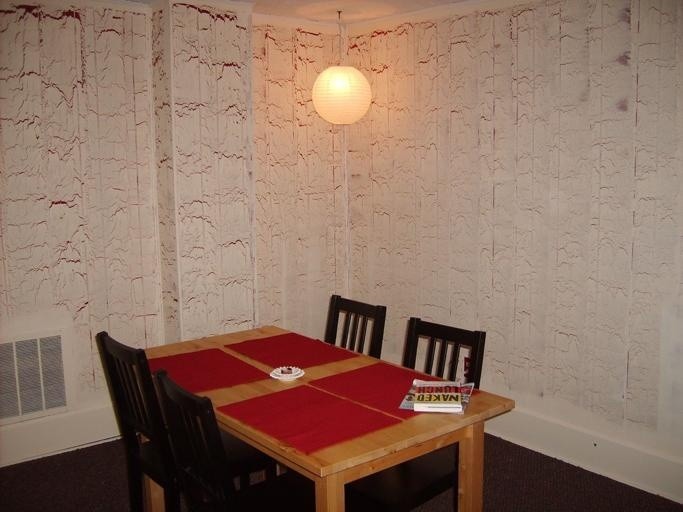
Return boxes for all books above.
[412,380,462,412]
[398,378,474,415]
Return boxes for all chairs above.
[321,294,386,362]
[343,316,486,511]
[95,330,281,511]
[149,368,315,511]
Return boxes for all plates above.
[265,365,305,382]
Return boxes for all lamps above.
[309,9,372,127]
